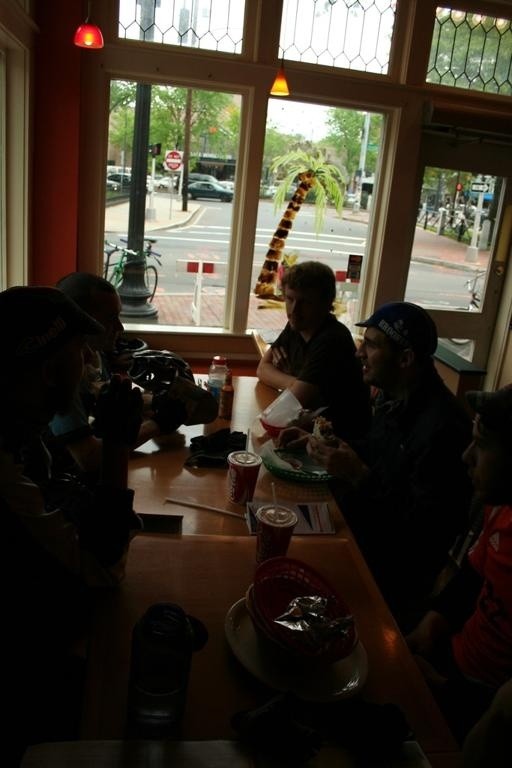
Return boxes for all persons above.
[256,260,374,448]
[1,283,142,768]
[55,272,187,474]
[275,300,475,637]
[398,382,511,767]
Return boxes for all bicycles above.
[448,271,488,345]
[102,234,160,305]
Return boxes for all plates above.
[225,598,369,701]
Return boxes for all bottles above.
[207,356,230,400]
[217,372,235,421]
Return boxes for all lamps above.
[270,52,290,96]
[74,0,104,49]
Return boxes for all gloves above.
[93,373,143,446]
[148,390,190,436]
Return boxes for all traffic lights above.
[455,182,462,192]
[147,142,162,156]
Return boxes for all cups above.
[225,452,261,509]
[254,505,297,565]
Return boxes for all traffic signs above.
[470,182,490,193]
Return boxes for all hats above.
[466,383,512,426]
[1,286,107,340]
[357,302,437,357]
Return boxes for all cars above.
[106,165,356,209]
[436,203,490,223]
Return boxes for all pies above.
[313,416,338,446]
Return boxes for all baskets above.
[263,448,332,481]
[254,557,359,664]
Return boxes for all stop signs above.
[164,151,183,170]
